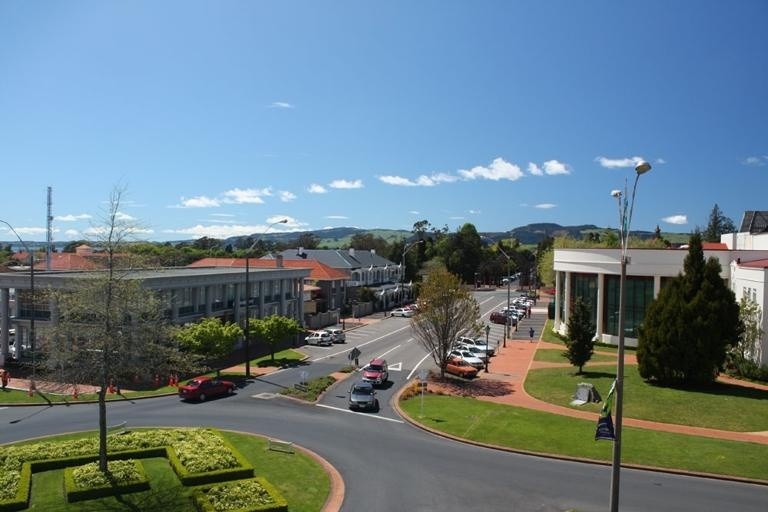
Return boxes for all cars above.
[179,376,238,402]
[445,337,495,378]
[348,358,389,412]
[304,328,345,347]
[489,294,540,326]
[390,304,419,317]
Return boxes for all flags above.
[595,380,618,441]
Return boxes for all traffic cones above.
[133,372,140,384]
[109,384,113,393]
[74,389,78,399]
[155,374,159,386]
[29,388,32,396]
[169,373,178,386]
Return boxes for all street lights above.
[246,219,288,376]
[610,160,651,512]
[401,240,423,307]
[480,236,510,338]
[483,325,490,372]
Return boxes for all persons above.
[0,370,11,388]
[527,327,536,343]
